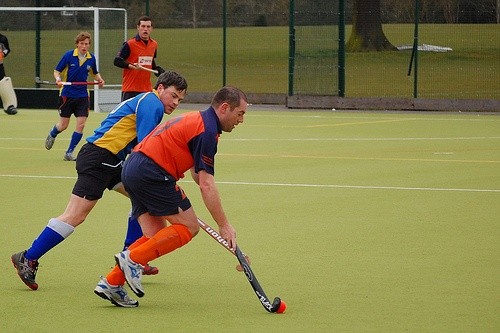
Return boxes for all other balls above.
[277,301,287,313]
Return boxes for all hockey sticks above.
[233,227,280,313]
[195,217,251,271]
[33,78,111,87]
[134,63,162,74]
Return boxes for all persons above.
[44,32,105,161]
[113,15,166,104]
[0,33,18,115]
[94,86,247,308]
[10,72,188,290]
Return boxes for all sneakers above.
[94,275,139,307]
[63,152,77,161]
[114,250,146,297]
[4,104,17,114]
[11,250,39,290]
[45,133,55,150]
[142,263,159,275]
